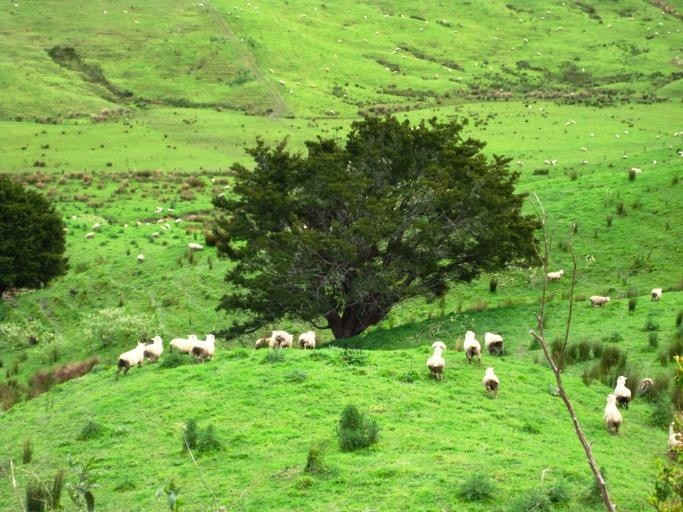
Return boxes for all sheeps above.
[426,341,446,381]
[253,329,318,351]
[116,340,148,382]
[482,367,500,398]
[62,203,207,263]
[463,330,481,365]
[614,376,631,411]
[668,421,683,451]
[590,295,611,305]
[484,331,505,357]
[546,269,565,282]
[143,334,215,364]
[603,394,622,434]
[649,287,664,301]
